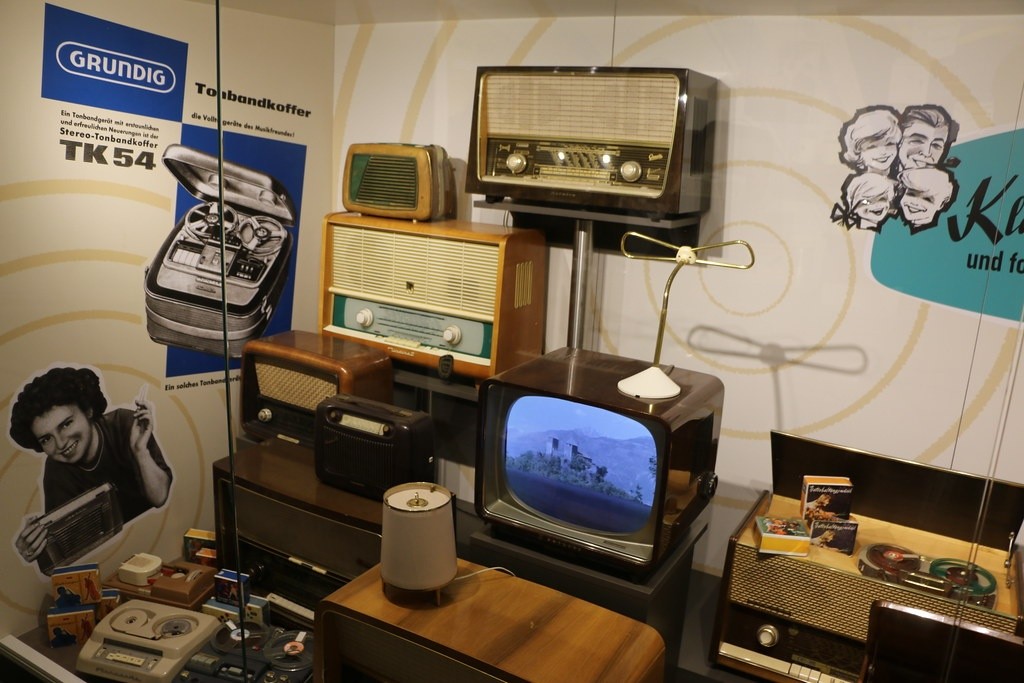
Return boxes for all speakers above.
[341,142,453,222]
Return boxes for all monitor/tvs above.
[474,348,725,575]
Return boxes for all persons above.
[13,359,176,565]
